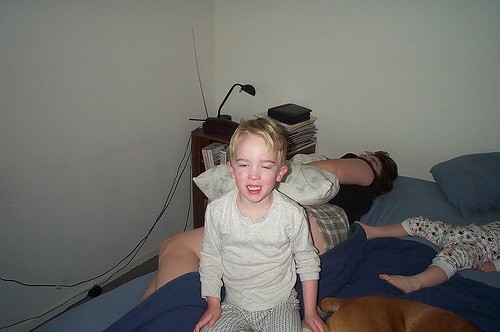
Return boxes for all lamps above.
[218,83,255,120]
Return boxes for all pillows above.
[191,154,340,206]
[430,152,500,220]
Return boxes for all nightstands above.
[190,128,314,228]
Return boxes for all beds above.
[32,175,500,332]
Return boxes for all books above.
[201,106,318,171]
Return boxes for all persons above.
[134,148,400,302]
[352,213,499,293]
[189,116,331,332]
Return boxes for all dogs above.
[320,297,483,332]
